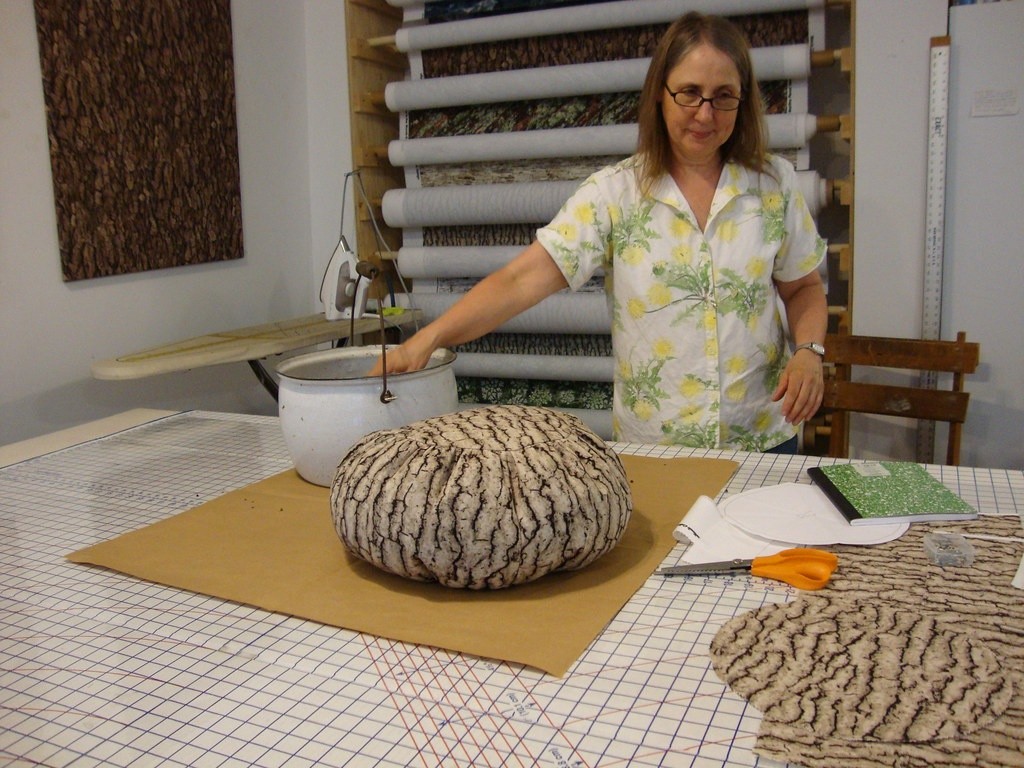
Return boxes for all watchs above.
[794,342,825,361]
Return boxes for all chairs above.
[822,325,979,466]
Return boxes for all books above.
[807,461,977,526]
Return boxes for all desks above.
[90,308,424,402]
[0,406,1024,768]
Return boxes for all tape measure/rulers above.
[914,36,951,463]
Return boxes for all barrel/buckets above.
[275,261,458,488]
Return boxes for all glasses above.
[663,80,745,111]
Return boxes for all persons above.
[369,11,829,454]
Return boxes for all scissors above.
[653,548,837,591]
